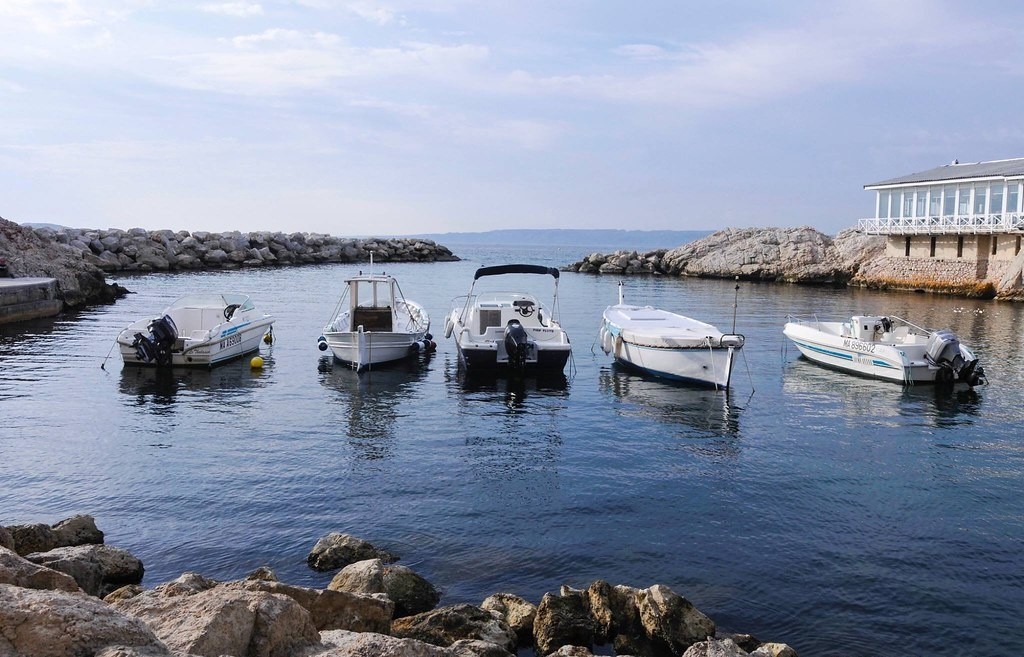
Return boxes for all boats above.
[599,279,745,393]
[783,312,986,389]
[444,263,573,379]
[117,293,277,369]
[322,250,431,374]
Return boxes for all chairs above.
[880,326,909,344]
[190,330,206,339]
